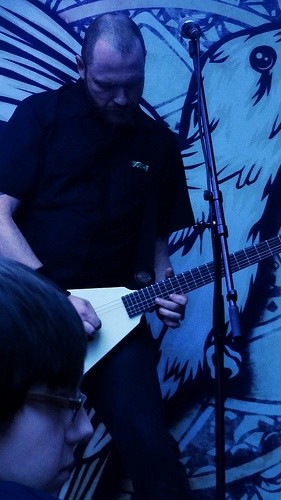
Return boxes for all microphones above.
[178,16,201,40]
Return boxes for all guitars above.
[66,233,281,377]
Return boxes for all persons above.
[0,257,95,500]
[0,16,195,500]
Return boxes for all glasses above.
[27,392,87,423]
